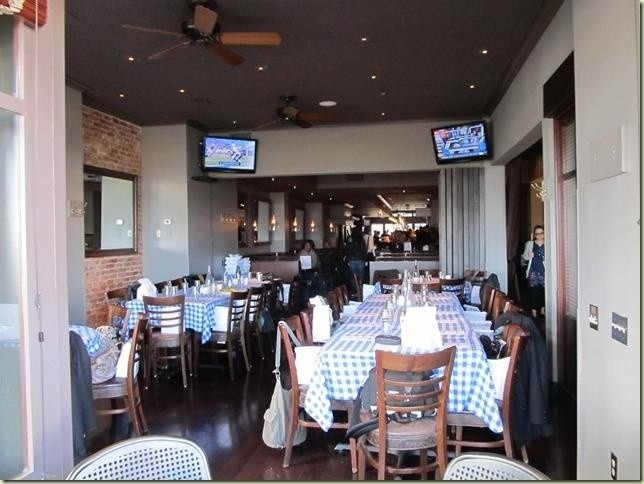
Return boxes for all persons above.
[341,227,367,296]
[299,240,317,271]
[363,226,438,261]
[230,142,242,166]
[518,224,546,324]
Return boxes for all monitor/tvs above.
[429,120,494,165]
[200,133,257,174]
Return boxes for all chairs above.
[107,272,296,388]
[439,451,551,482]
[348,344,457,482]
[60,433,215,480]
[90,318,151,438]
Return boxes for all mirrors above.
[293,206,306,242]
[83,163,139,259]
[254,195,271,245]
[236,191,248,249]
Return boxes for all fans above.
[249,94,339,131]
[119,2,281,65]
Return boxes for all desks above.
[243,245,444,284]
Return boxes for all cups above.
[381,283,399,334]
[419,270,432,282]
[222,270,263,289]
[160,277,200,299]
[420,282,432,308]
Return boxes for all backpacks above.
[346,366,439,440]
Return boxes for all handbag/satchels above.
[261,379,307,449]
[115,337,141,379]
[367,252,375,261]
[254,306,275,333]
[312,305,333,343]
[91,341,124,384]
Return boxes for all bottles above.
[413,259,419,277]
[208,275,218,297]
[401,269,416,308]
[203,264,213,286]
